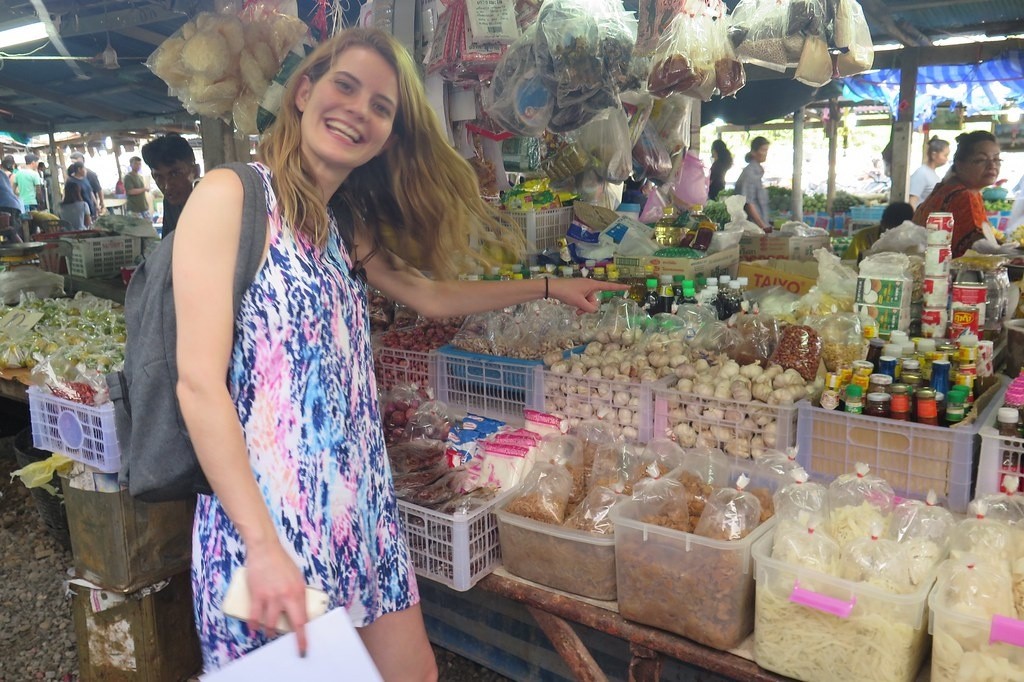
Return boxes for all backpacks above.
[104,161,355,502]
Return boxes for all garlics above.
[543,326,825,460]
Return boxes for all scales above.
[0,241,48,272]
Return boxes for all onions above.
[367,293,461,440]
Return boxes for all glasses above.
[963,154,1004,168]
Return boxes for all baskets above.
[32,230,141,280]
[469,205,575,255]
[25,384,123,473]
[369,321,1024,592]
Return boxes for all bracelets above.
[544,276,549,300]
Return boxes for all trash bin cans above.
[14,424,70,545]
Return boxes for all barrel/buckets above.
[120,265,136,285]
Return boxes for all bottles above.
[681,221,717,253]
[861,315,879,340]
[997,368,1024,476]
[622,265,749,322]
[458,264,620,287]
[982,267,1009,343]
[817,330,978,428]
[688,205,710,223]
[655,207,682,246]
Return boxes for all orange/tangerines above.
[0,300,126,378]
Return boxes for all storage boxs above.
[0,199,1024,682]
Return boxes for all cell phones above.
[221,566,330,633]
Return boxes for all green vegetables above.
[703,201,730,230]
[765,185,792,196]
[717,189,734,199]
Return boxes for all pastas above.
[755,500,937,682]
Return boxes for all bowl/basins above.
[982,188,1008,200]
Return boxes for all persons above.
[142,133,200,239]
[867,158,890,184]
[172,27,631,681]
[42,153,66,215]
[70,151,105,222]
[38,161,50,210]
[842,202,914,272]
[733,137,772,228]
[58,181,93,232]
[68,161,99,219]
[708,140,733,201]
[909,135,951,212]
[9,153,44,235]
[124,157,155,226]
[0,154,23,243]
[913,130,1004,258]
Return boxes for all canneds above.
[922,212,988,347]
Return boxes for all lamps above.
[0,12,61,50]
